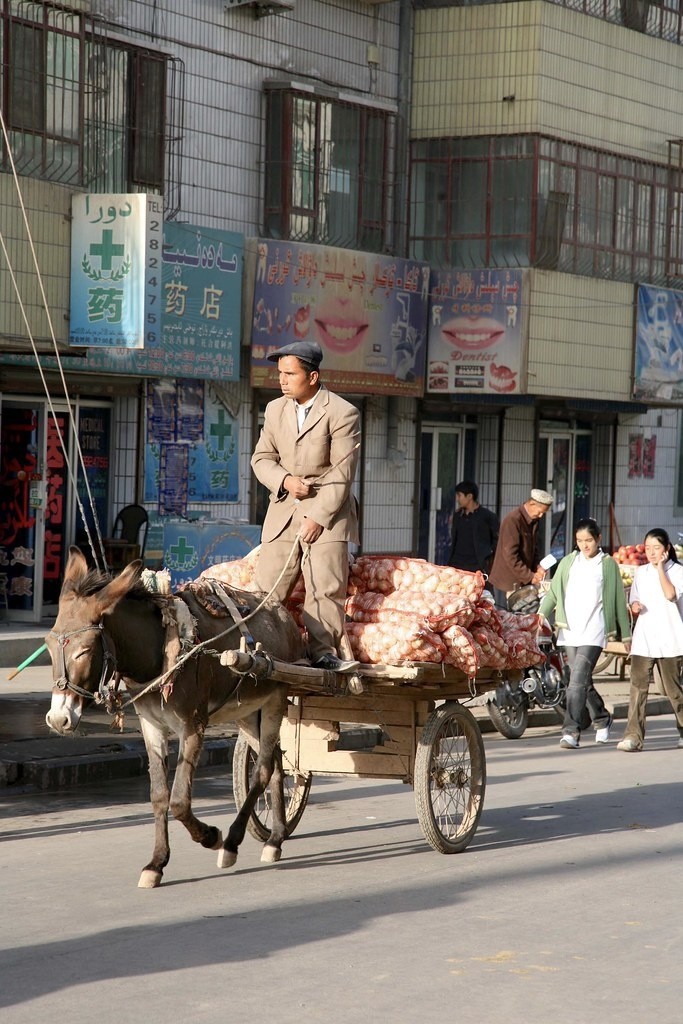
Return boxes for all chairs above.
[97,504,149,581]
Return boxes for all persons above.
[537,517,635,746]
[450,478,499,590]
[235,340,360,693]
[487,489,556,608]
[616,526,683,752]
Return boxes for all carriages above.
[44,545,523,889]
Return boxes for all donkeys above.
[43,546,307,887]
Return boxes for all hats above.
[266,341,323,367]
[530,488,554,506]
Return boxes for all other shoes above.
[311,652,360,674]
[616,738,637,751]
[677,736,683,747]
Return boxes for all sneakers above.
[560,734,580,748]
[595,713,613,743]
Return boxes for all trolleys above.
[591,585,683,696]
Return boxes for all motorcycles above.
[479,554,592,739]
